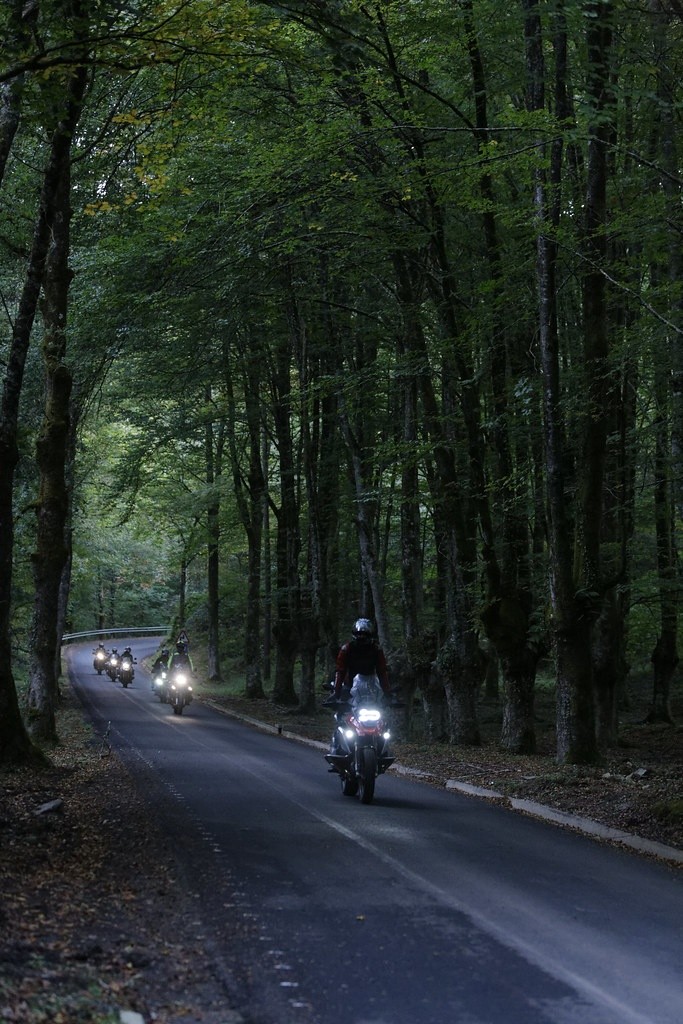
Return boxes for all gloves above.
[326,693,339,702]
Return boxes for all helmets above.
[350,618,375,644]
[112,646,118,653]
[162,649,169,656]
[124,646,131,653]
[176,641,185,651]
[99,642,105,648]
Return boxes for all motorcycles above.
[92,648,110,674]
[153,663,169,704]
[166,671,194,714]
[316,679,398,804]
[118,656,137,687]
[106,654,120,682]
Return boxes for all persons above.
[327,617,395,774]
[93,640,194,680]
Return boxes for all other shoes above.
[328,764,338,773]
